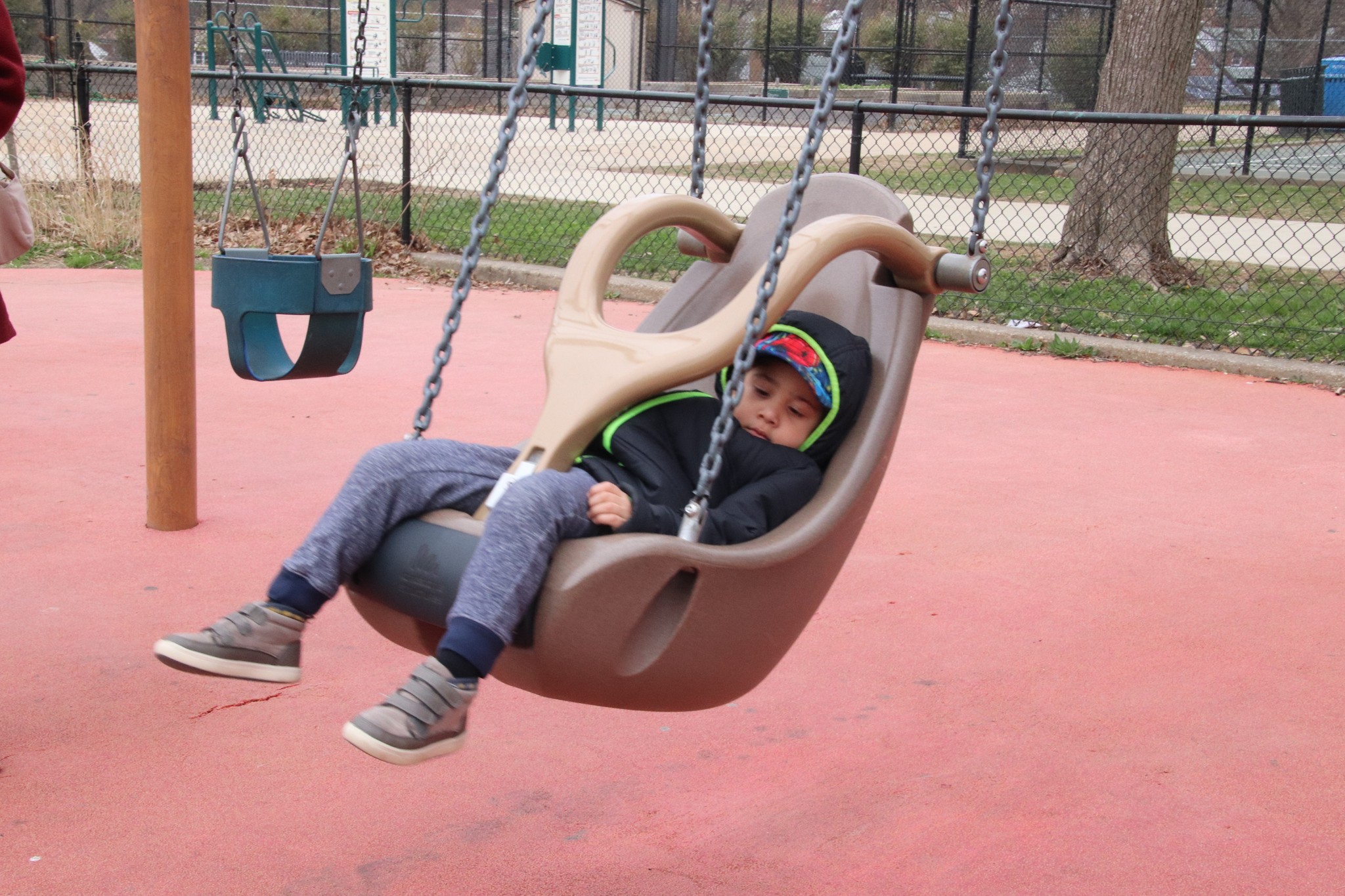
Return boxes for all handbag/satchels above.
[0,160,35,264]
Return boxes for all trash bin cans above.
[1277,65,1328,137]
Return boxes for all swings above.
[338,0,1016,715]
[209,1,375,383]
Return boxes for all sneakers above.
[153,606,305,683]
[341,654,479,766]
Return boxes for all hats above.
[752,331,834,408]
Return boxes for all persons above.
[0,1,27,344]
[154,310,872,766]
